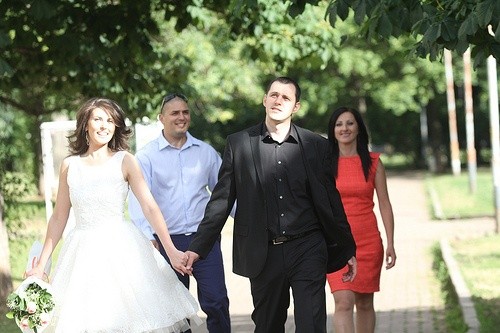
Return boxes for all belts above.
[268,232,311,245]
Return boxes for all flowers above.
[6,240,57,333]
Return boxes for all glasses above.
[160,93,188,111]
